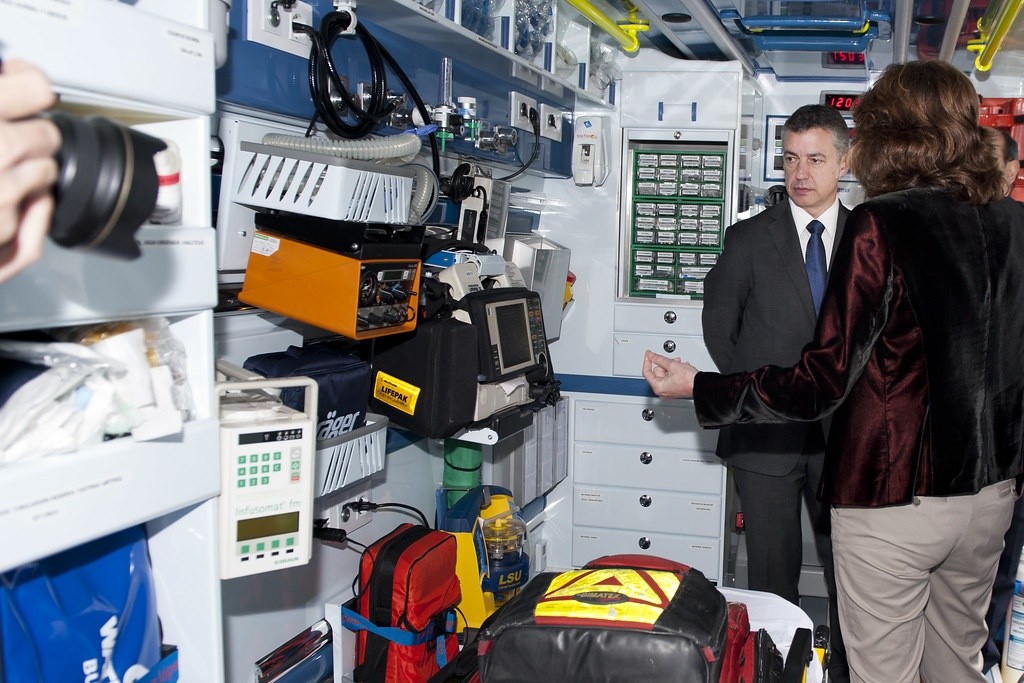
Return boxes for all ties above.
[806,220,828,319]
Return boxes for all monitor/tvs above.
[486,299,536,377]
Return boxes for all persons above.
[642,62,1024,683]
[703,105,851,681]
[983,126,1024,683]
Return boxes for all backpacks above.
[479,554,784,683]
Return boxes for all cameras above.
[40,110,168,260]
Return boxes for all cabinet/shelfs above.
[572,399,725,585]
[608,304,722,381]
